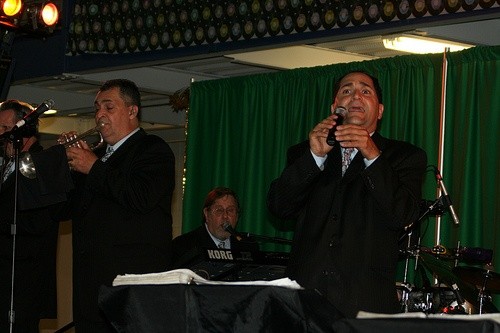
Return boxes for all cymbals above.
[452,265,500,292]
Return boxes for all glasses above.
[208,207,238,216]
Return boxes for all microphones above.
[14,98,56,130]
[434,167,459,224]
[326,105,347,146]
[223,221,242,241]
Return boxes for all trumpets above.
[18,121,106,180]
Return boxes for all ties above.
[341,147,355,177]
[101,147,115,162]
[3,161,14,181]
[218,242,225,249]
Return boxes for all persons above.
[51,78,176,332]
[168,186,264,269]
[267,71,427,333]
[0,98,60,333]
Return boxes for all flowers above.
[167,87,190,112]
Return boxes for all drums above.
[394,284,489,316]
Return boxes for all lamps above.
[1,0,59,35]
[382,34,477,54]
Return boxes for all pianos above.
[171,250,291,278]
